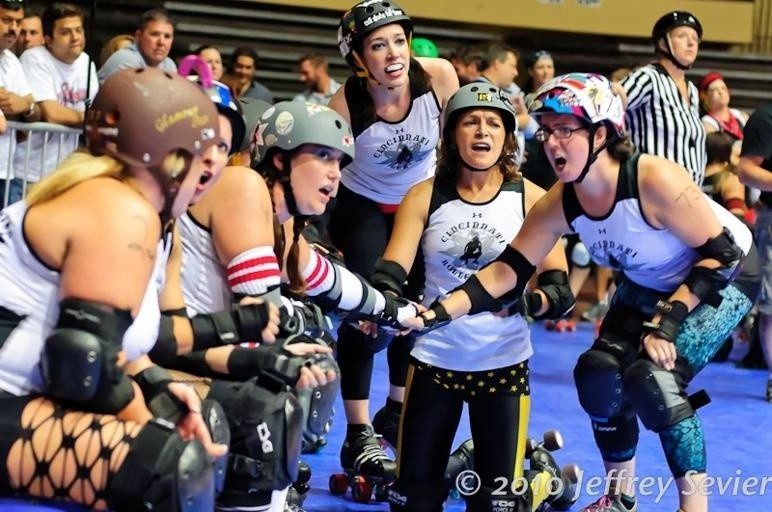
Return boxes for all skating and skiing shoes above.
[452,439,475,492]
[372,405,407,462]
[329,423,399,503]
[579,489,637,512]
[544,305,576,332]
[526,430,583,510]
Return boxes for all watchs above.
[25,95,34,119]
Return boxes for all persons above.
[521,51,553,82]
[618,11,706,186]
[0,4,42,208]
[13,4,98,200]
[228,48,274,107]
[295,51,343,102]
[180,98,424,343]
[0,71,227,509]
[121,80,333,509]
[100,37,134,67]
[737,100,770,400]
[195,44,225,87]
[98,11,178,81]
[700,72,749,132]
[482,48,537,169]
[450,44,487,80]
[371,84,575,511]
[325,2,460,485]
[18,13,47,52]
[424,72,760,508]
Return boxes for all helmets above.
[529,73,625,138]
[409,38,438,58]
[85,66,220,168]
[653,11,703,52]
[444,82,517,133]
[337,0,413,60]
[249,101,355,170]
[182,76,245,155]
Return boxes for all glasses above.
[535,124,589,142]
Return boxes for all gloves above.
[379,293,424,331]
[135,367,199,437]
[638,301,688,359]
[367,260,408,298]
[228,338,334,388]
[396,300,451,347]
[298,301,322,332]
[191,300,269,346]
[495,289,540,317]
